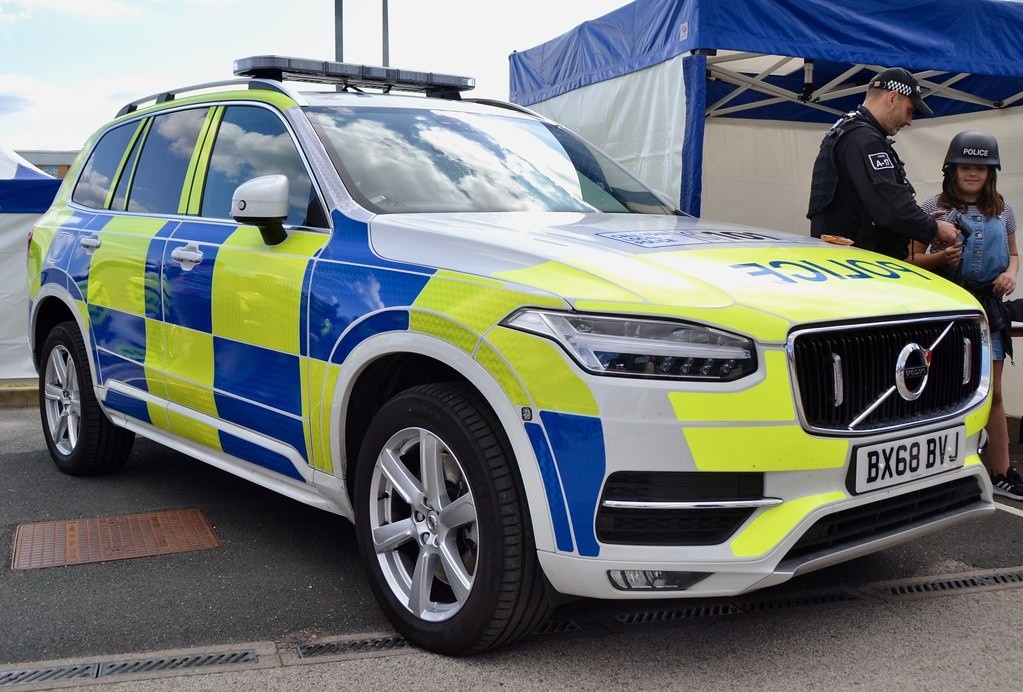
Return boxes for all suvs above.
[26,55,998,662]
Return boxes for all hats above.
[869,67,933,115]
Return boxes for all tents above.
[508,0,1023,421]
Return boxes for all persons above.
[905,131,1023,501]
[805,67,958,262]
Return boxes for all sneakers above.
[991,466,1023,500]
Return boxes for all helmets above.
[943,130,1001,170]
